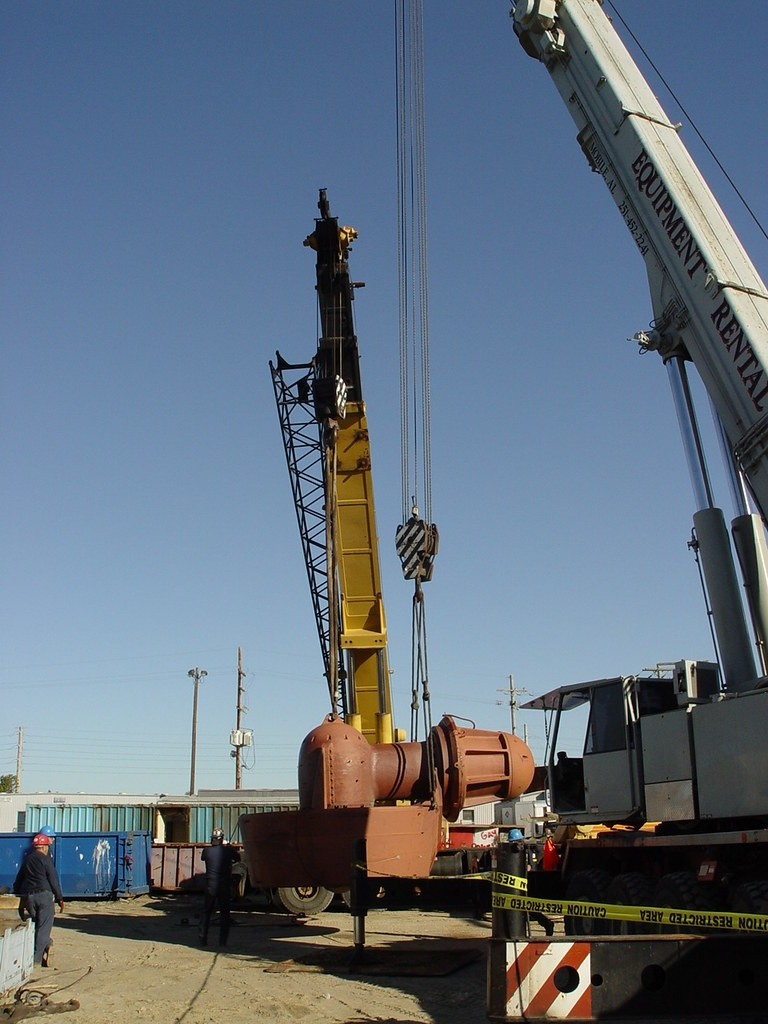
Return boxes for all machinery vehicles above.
[237,1,766,1024]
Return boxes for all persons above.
[201,827,241,948]
[14,827,63,964]
[508,829,555,937]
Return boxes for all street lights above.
[187,668,208,800]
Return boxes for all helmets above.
[211,829,224,837]
[39,826,57,836]
[32,834,50,844]
[506,829,524,841]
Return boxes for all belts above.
[30,889,48,895]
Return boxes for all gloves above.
[58,900,64,913]
